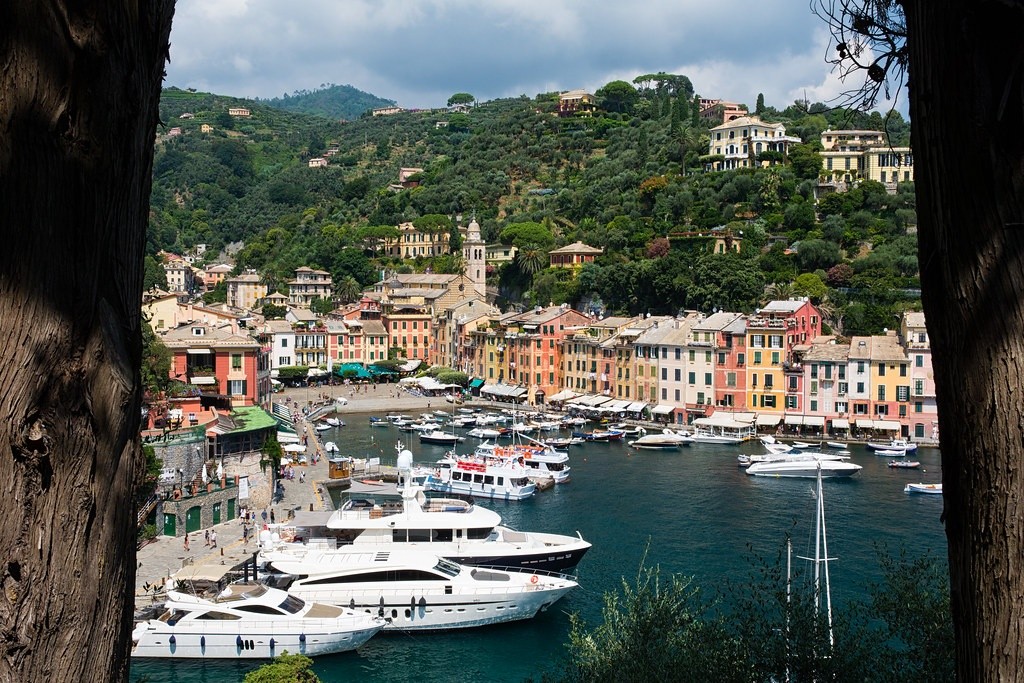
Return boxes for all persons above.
[270,509,275,524]
[311,449,321,464]
[277,480,285,490]
[279,396,298,408]
[184,535,189,551]
[293,411,299,423]
[251,512,256,525]
[302,435,308,445]
[204,530,210,544]
[303,426,307,434]
[261,509,268,521]
[209,529,217,547]
[240,508,249,522]
[343,378,360,396]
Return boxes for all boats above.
[873,448,907,457]
[888,459,921,469]
[129,579,390,659]
[826,441,848,449]
[866,438,918,453]
[903,483,943,495]
[222,394,944,636]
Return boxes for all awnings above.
[551,391,676,414]
[271,370,293,378]
[467,376,526,397]
[307,368,327,376]
[755,414,902,431]
